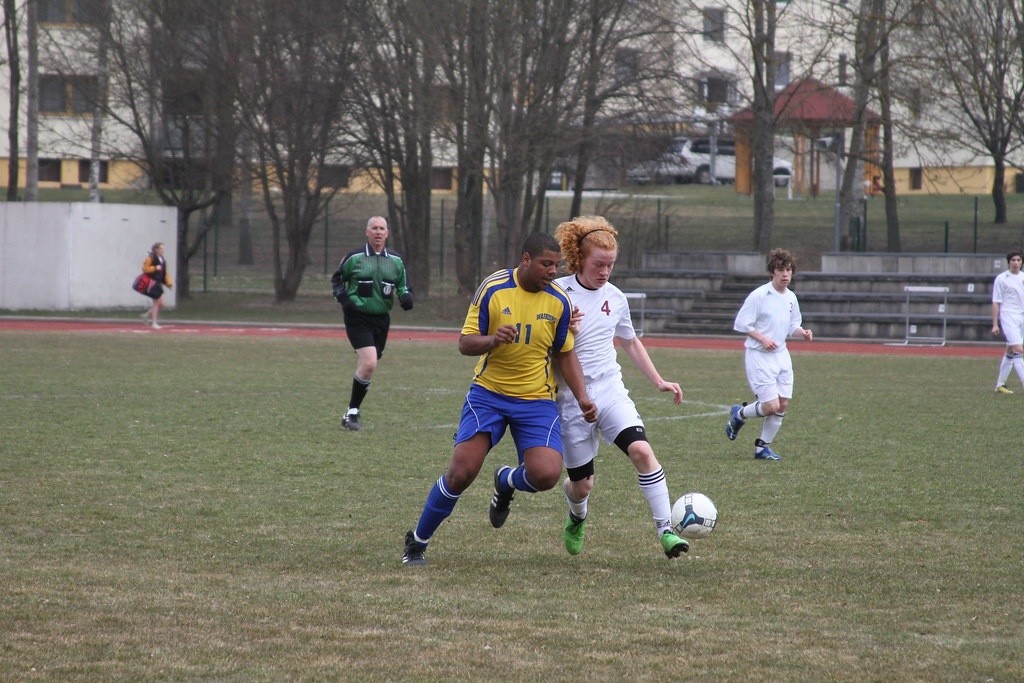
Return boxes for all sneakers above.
[726,401,748,440]
[563,509,588,554]
[996,384,1014,395]
[489,465,516,527]
[342,407,361,430]
[402,530,430,566]
[754,439,782,460]
[661,529,689,559]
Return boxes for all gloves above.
[336,293,358,313]
[398,292,413,311]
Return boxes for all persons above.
[402,232,598,565]
[992,250,1024,394]
[331,216,414,431]
[138,242,174,329]
[725,248,813,460]
[548,215,689,559]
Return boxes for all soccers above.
[671,493,719,538]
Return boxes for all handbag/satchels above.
[133,273,163,300]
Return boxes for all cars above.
[626,136,793,187]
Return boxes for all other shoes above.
[151,321,161,329]
[141,314,150,322]
